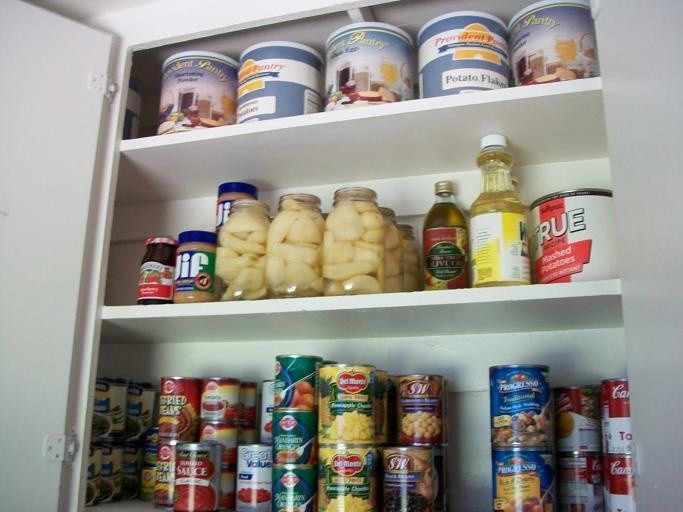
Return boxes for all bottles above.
[468,135,532,289]
[216,182,257,230]
[173,231,218,302]
[421,180,469,290]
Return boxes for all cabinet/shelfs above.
[1,0,641,512]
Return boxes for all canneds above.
[213,186,422,307]
[136,235,181,304]
[85,353,450,512]
[489,364,636,512]
